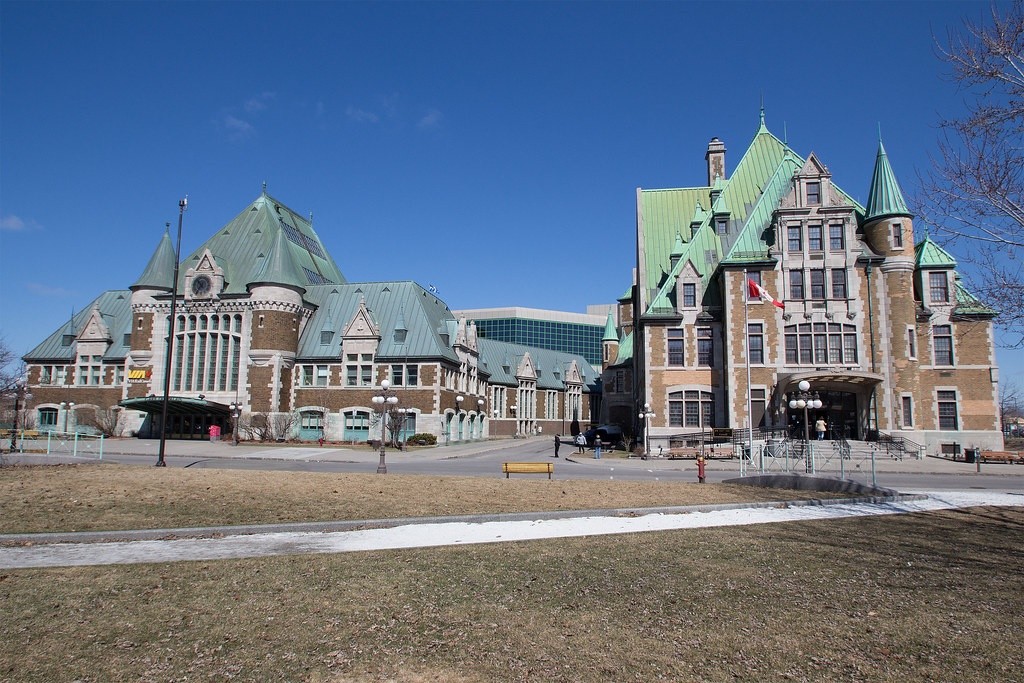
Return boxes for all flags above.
[747,278,785,310]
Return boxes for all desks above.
[602,442,611,452]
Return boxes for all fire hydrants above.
[318,437,325,448]
[696,456,708,484]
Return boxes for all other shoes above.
[555,456,559,458]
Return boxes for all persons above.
[554,432,561,458]
[816,416,827,441]
[594,435,602,459]
[577,432,587,454]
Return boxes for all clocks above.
[193,277,210,295]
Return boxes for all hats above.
[819,416,824,419]
[556,434,560,436]
[579,432,582,434]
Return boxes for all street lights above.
[372,379,398,474]
[638,403,657,456]
[156,194,189,468]
[789,380,822,474]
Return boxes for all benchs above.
[668,448,699,460]
[0,430,11,439]
[980,451,1018,465]
[610,445,616,452]
[502,462,554,479]
[705,448,733,460]
[17,432,43,440]
[1017,452,1024,464]
